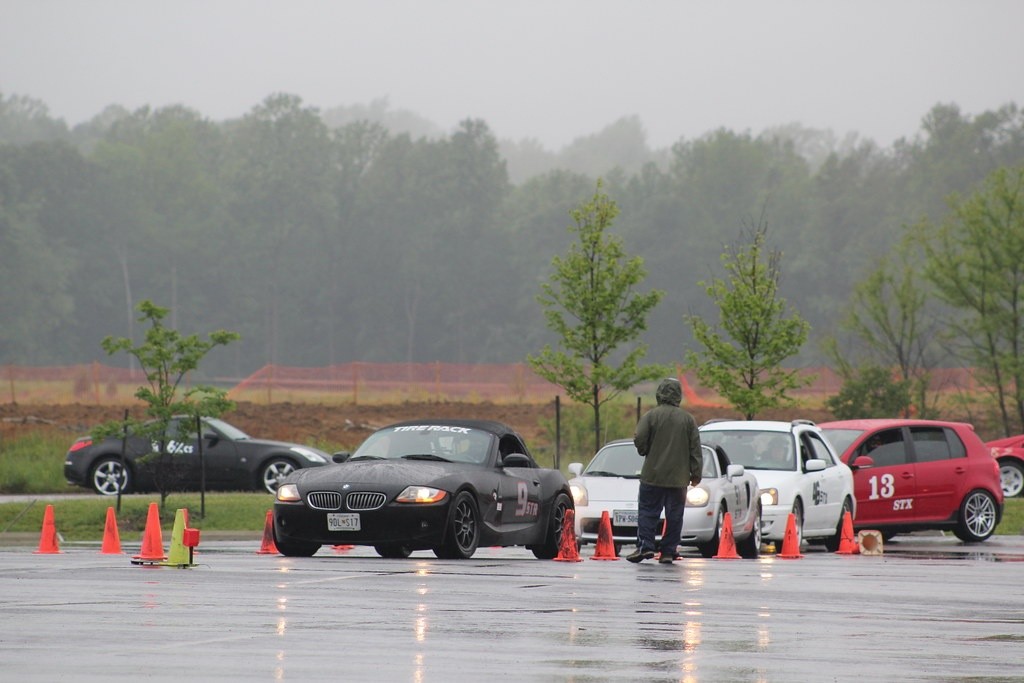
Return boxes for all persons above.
[763,438,794,470]
[627,377,704,564]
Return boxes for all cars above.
[986,434,1024,498]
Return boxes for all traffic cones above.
[858,529,884,556]
[834,512,858,555]
[708,512,743,560]
[256,509,279,555]
[99,506,126,554]
[588,511,621,560]
[775,513,804,559]
[32,504,64,554]
[553,509,581,562]
[132,502,169,561]
[655,513,682,560]
[154,508,199,569]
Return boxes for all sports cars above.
[274,417,576,561]
[62,413,335,496]
[566,437,764,559]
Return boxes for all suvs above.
[696,419,858,552]
[805,419,1005,544]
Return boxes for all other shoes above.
[659,552,672,563]
[626,549,654,563]
